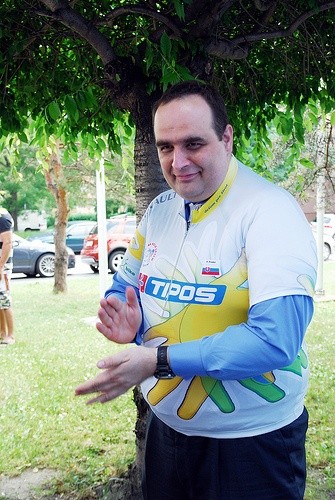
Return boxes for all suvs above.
[80,214,135,275]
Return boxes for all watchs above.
[153,346,175,380]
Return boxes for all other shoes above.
[2,336,15,345]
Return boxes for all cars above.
[16,209,49,233]
[11,234,76,278]
[308,213,335,262]
[25,220,97,253]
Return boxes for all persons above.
[75,80,316,500]
[0,205,13,345]
